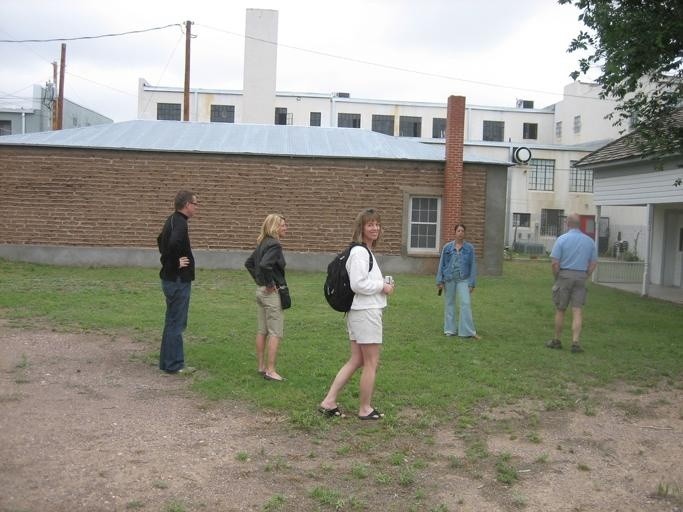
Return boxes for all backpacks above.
[323,241,374,312]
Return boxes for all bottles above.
[437,287,442,296]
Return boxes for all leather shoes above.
[257,367,288,383]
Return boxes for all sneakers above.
[570,340,585,353]
[547,338,563,350]
[165,364,196,374]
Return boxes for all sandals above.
[319,404,346,420]
[358,409,385,422]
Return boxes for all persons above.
[244,212,291,381]
[317,208,395,420]
[156,189,198,375]
[435,224,481,340]
[545,211,598,353]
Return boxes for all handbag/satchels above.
[278,286,292,309]
